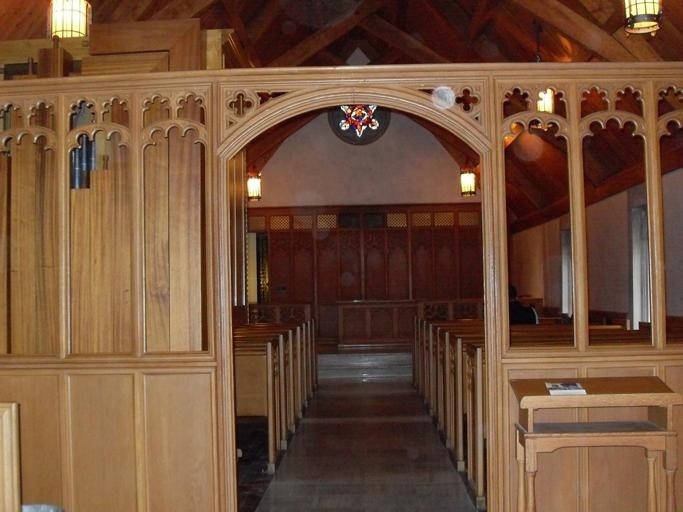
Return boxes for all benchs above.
[231,312,321,477]
[411,308,683,512]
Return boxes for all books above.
[545,382,587,395]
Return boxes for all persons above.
[508,284,539,324]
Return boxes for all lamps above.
[246,164,262,204]
[40,0,93,60]
[525,87,557,134]
[457,155,477,198]
[619,0,664,38]
